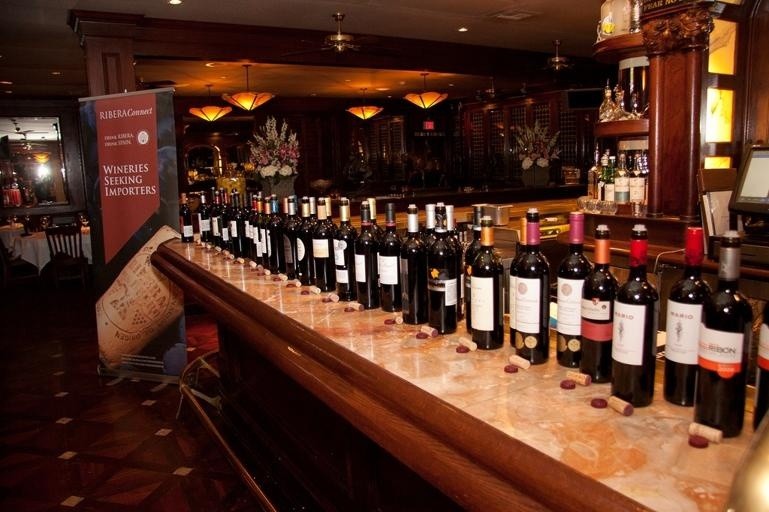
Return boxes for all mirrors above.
[0,110,88,214]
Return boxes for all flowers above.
[248,117,300,178]
[516,119,562,171]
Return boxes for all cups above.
[685,227,704,265]
[40,217,49,231]
[79,213,90,228]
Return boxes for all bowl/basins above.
[472,203,514,225]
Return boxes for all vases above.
[522,170,549,188]
[261,179,297,200]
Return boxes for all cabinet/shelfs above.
[584,0,714,248]
[307,201,582,238]
[150,238,769,512]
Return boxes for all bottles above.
[225,193,234,251]
[464,204,488,336]
[180,193,194,243]
[283,195,302,279]
[211,191,224,248]
[296,196,313,285]
[249,194,260,263]
[557,212,595,369]
[333,200,358,302]
[264,197,276,271]
[425,206,435,240]
[470,216,505,350]
[599,156,613,201]
[309,196,317,221]
[630,149,646,205]
[694,232,754,438]
[312,198,338,293]
[210,187,216,211]
[598,85,616,122]
[377,202,404,313]
[197,191,212,241]
[511,218,528,350]
[229,192,242,259]
[612,90,631,120]
[752,299,769,436]
[428,202,458,335]
[325,197,339,230]
[270,194,285,274]
[611,225,660,409]
[367,198,385,239]
[580,224,618,383]
[399,205,428,325]
[614,154,631,203]
[283,197,289,219]
[253,201,264,265]
[515,209,550,365]
[443,204,464,322]
[663,227,714,409]
[354,201,378,309]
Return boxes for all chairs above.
[45,226,89,284]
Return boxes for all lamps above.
[222,64,275,111]
[188,84,232,121]
[346,88,384,120]
[404,73,448,109]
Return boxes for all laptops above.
[729,146,769,213]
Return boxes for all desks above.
[12,227,92,274]
[3,188,37,207]
[0,223,25,252]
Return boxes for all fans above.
[283,11,375,55]
[544,36,574,71]
